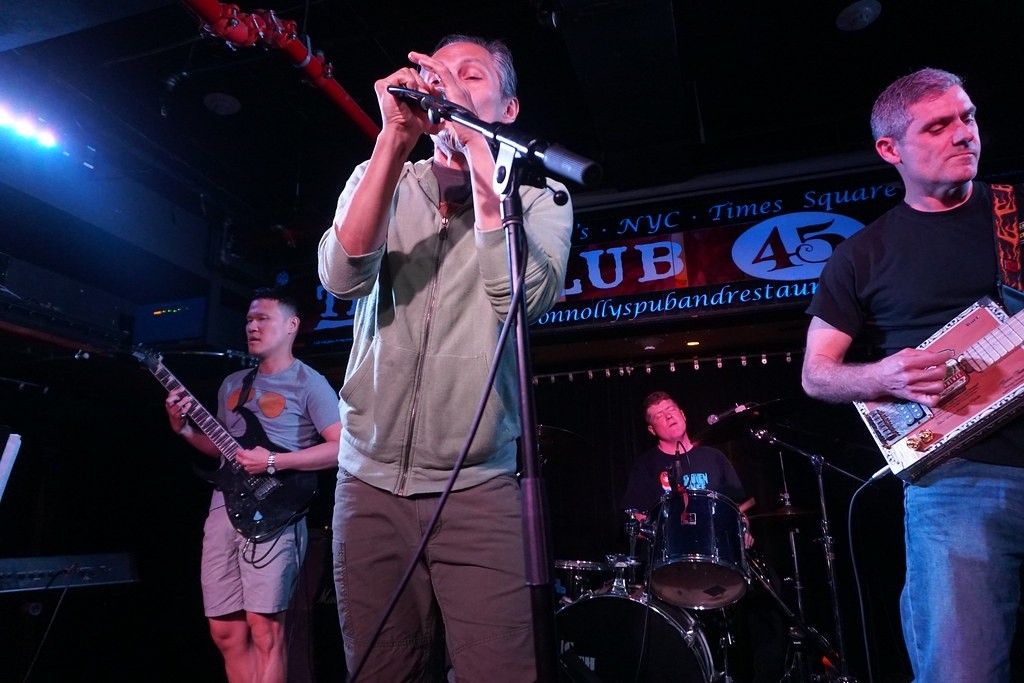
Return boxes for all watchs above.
[266,452,277,474]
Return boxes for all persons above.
[163,288,341,683]
[623,392,786,683]
[801,69,1024,683]
[316,32,574,683]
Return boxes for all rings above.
[181,414,185,417]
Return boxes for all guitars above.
[130,340,321,544]
[851,292,1024,489]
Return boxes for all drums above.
[554,559,608,609]
[643,487,751,612]
[556,586,716,683]
[605,554,645,589]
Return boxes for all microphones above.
[705,404,745,425]
[408,84,446,111]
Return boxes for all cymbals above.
[697,395,799,447]
[532,422,587,457]
